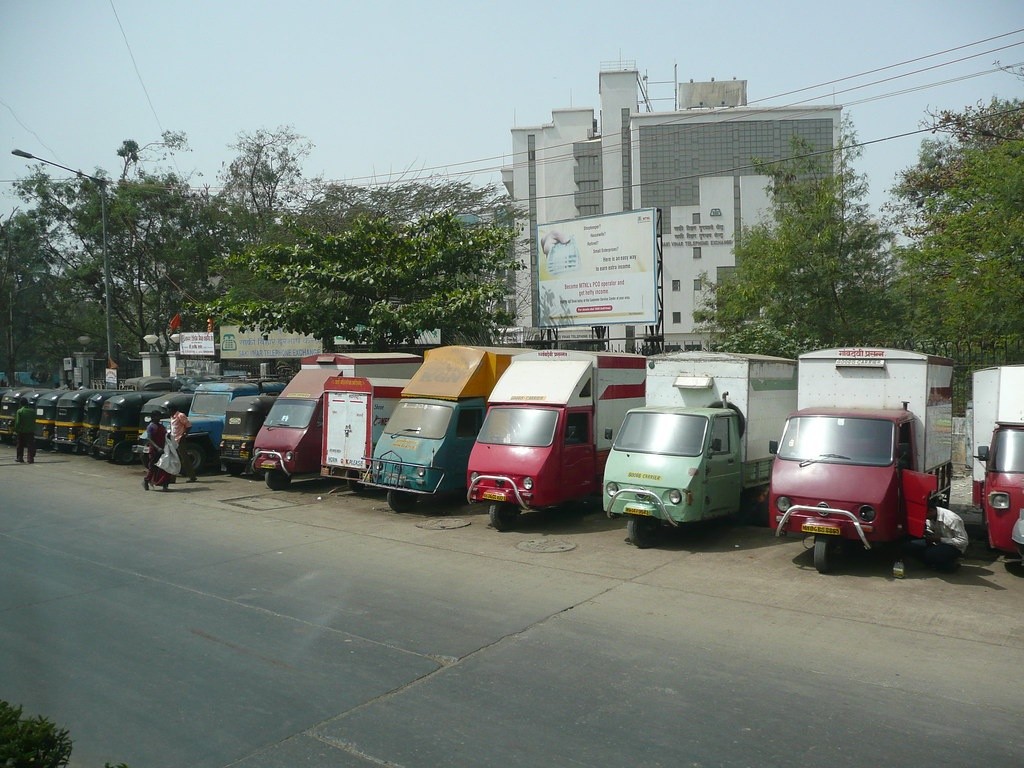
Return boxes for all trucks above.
[356,345,537,512]
[467,348,646,532]
[0,371,289,476]
[319,375,413,495]
[600,350,797,548]
[249,352,422,490]
[767,343,953,576]
[970,364,1023,572]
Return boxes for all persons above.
[909,503,969,568]
[143,403,197,492]
[14,397,36,463]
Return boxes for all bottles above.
[892,558,905,578]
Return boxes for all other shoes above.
[186,478,197,483]
[143,477,149,490]
[15,458,24,463]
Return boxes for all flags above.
[171,314,179,330]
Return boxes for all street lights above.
[10,148,111,369]
